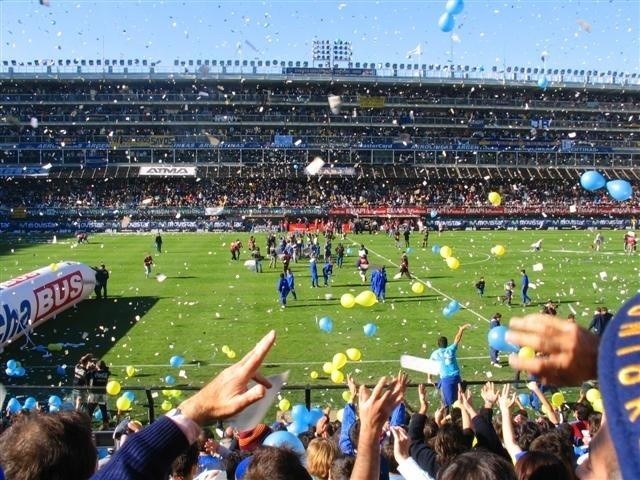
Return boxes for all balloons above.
[346,347,362,362]
[24,396,37,410]
[161,386,171,395]
[262,430,307,463]
[48,342,54,350]
[309,371,317,380]
[165,376,175,384]
[222,344,229,352]
[6,397,21,414]
[439,14,455,33]
[488,191,500,208]
[50,19,55,25]
[6,359,16,370]
[331,373,344,383]
[320,317,332,332]
[173,387,181,397]
[494,246,503,255]
[363,324,375,336]
[441,307,452,317]
[308,406,324,427]
[537,76,546,84]
[106,381,121,395]
[581,171,607,191]
[339,293,355,307]
[291,422,309,433]
[116,396,131,411]
[606,180,633,201]
[48,262,57,271]
[48,395,62,408]
[161,400,172,410]
[123,391,135,401]
[342,391,351,402]
[413,283,422,294]
[291,403,307,423]
[48,396,62,407]
[127,366,134,377]
[356,291,376,307]
[516,393,530,408]
[518,346,534,359]
[431,245,439,254]
[447,259,458,269]
[323,362,333,375]
[56,365,65,376]
[441,244,452,259]
[447,300,460,313]
[57,342,63,350]
[169,355,184,368]
[332,352,347,368]
[446,0,464,16]
[278,398,289,411]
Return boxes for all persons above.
[322,260,334,287]
[251,247,263,273]
[155,233,162,253]
[71,229,90,246]
[489,313,503,367]
[356,254,368,283]
[89,265,103,302]
[86,360,111,431]
[477,277,485,297]
[108,372,609,480]
[144,252,154,280]
[622,231,638,254]
[593,235,606,253]
[502,280,515,307]
[278,274,290,308]
[394,227,401,248]
[404,227,410,248]
[269,242,276,269]
[285,269,297,300]
[231,241,241,261]
[370,268,381,295]
[0,78,639,234]
[98,264,109,302]
[427,323,471,405]
[529,238,543,254]
[0,329,276,480]
[73,357,88,412]
[422,229,429,248]
[310,253,319,287]
[357,244,369,267]
[335,243,344,269]
[282,251,291,273]
[397,252,411,279]
[504,292,639,480]
[521,270,533,307]
[375,266,388,301]
[248,231,336,259]
